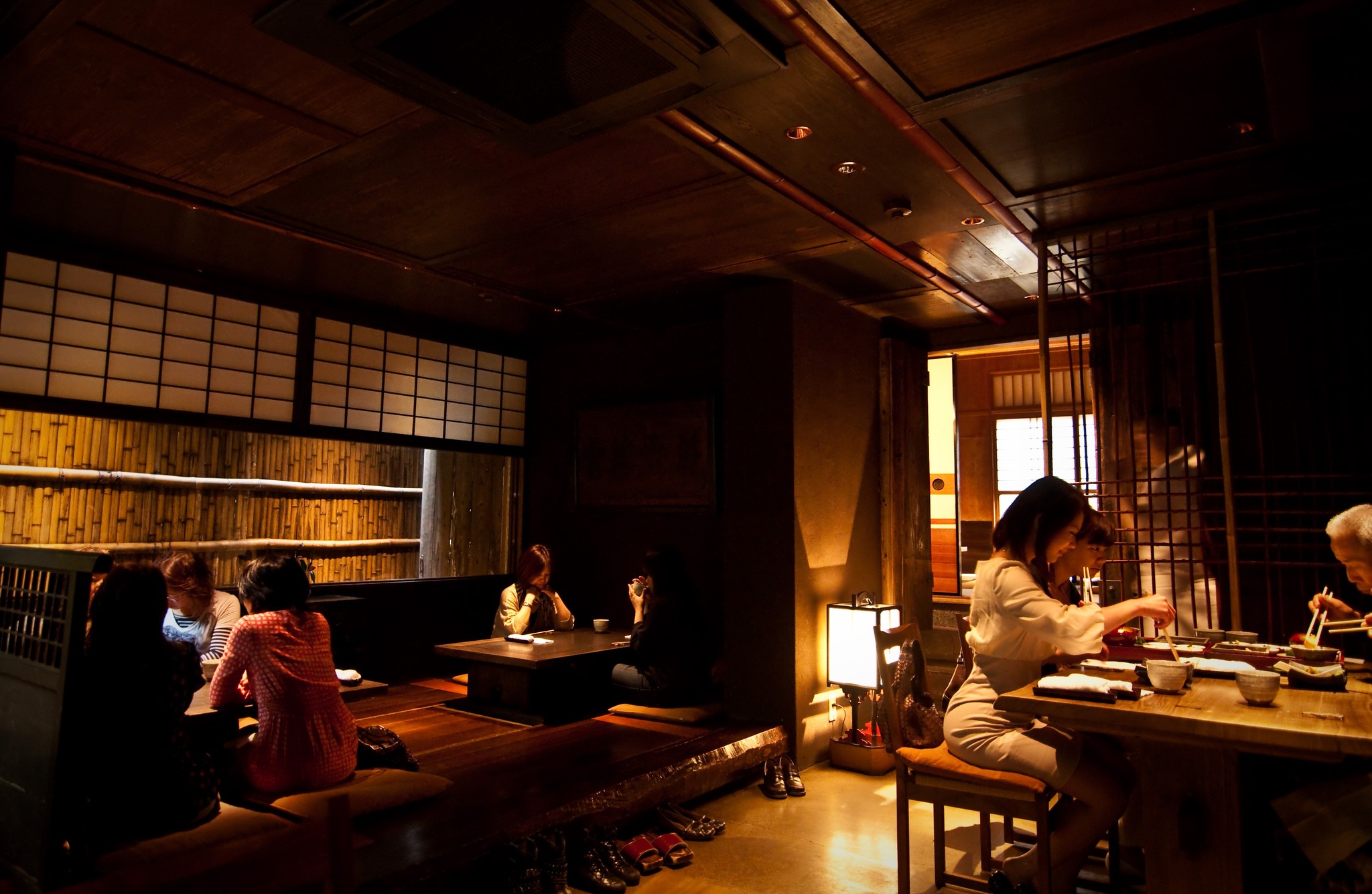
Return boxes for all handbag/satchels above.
[357,725,420,772]
[893,639,943,748]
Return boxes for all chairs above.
[871,623,1073,894]
[955,614,1123,894]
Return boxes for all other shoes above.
[1016,881,1036,894]
[988,870,1015,894]
[668,803,726,834]
[656,806,715,839]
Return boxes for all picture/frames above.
[571,391,718,514]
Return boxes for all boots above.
[511,839,543,894]
[543,834,568,894]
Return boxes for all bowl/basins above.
[1221,641,1280,655]
[1290,644,1339,662]
[1193,628,1225,643]
[1225,631,1258,644]
[1146,660,1187,694]
[1234,669,1281,705]
[1102,626,1140,646]
[202,659,221,679]
[633,580,646,596]
[1143,642,1206,652]
[593,619,609,632]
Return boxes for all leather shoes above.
[780,754,806,795]
[764,758,788,798]
[577,826,640,885]
[568,848,627,894]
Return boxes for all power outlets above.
[829,695,837,721]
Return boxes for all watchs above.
[523,602,532,608]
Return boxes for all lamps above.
[826,592,903,777]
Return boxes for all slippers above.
[646,828,694,864]
[615,834,664,871]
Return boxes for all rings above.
[630,592,635,595]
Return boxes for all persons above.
[612,542,725,709]
[153,549,240,674]
[1271,503,1372,879]
[491,545,575,639]
[942,475,1177,894]
[8,547,221,853]
[210,554,358,796]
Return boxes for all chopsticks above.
[1083,566,1094,604]
[1304,586,1334,646]
[1320,619,1372,633]
[1145,589,1180,662]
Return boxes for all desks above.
[184,679,389,805]
[993,659,1372,894]
[435,626,633,727]
[308,596,366,674]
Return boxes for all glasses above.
[167,592,187,604]
